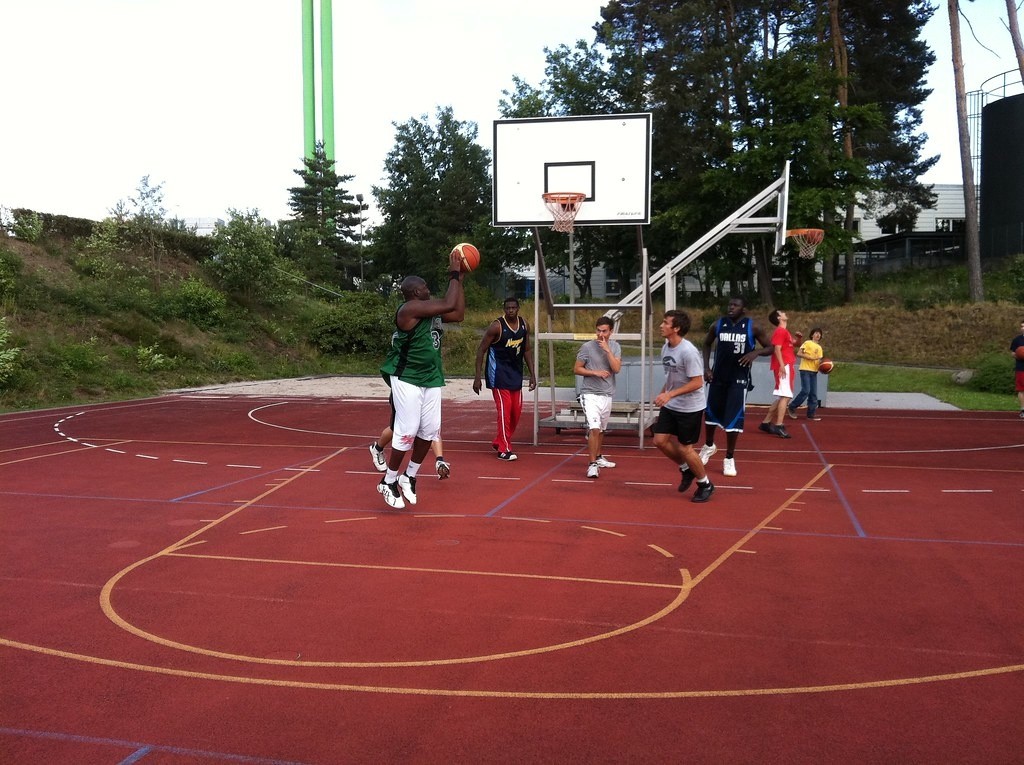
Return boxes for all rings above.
[456,260,460,262]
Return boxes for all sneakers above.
[595,455,616,468]
[376,475,405,508]
[758,421,775,434]
[435,460,450,481]
[774,423,791,439]
[492,446,498,451]
[723,457,737,476]
[397,470,417,505]
[678,465,696,493]
[699,442,718,466]
[787,405,798,419]
[586,463,600,478]
[806,415,822,421]
[497,452,517,460]
[691,480,715,503]
[370,442,387,472]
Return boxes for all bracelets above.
[449,270,459,282]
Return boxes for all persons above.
[574,317,621,478]
[369,388,451,481]
[787,328,823,421]
[698,295,774,476]
[1010,320,1024,418]
[376,248,465,508]
[473,297,536,460]
[759,309,802,438]
[652,310,714,503]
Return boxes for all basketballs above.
[449,242,481,273]
